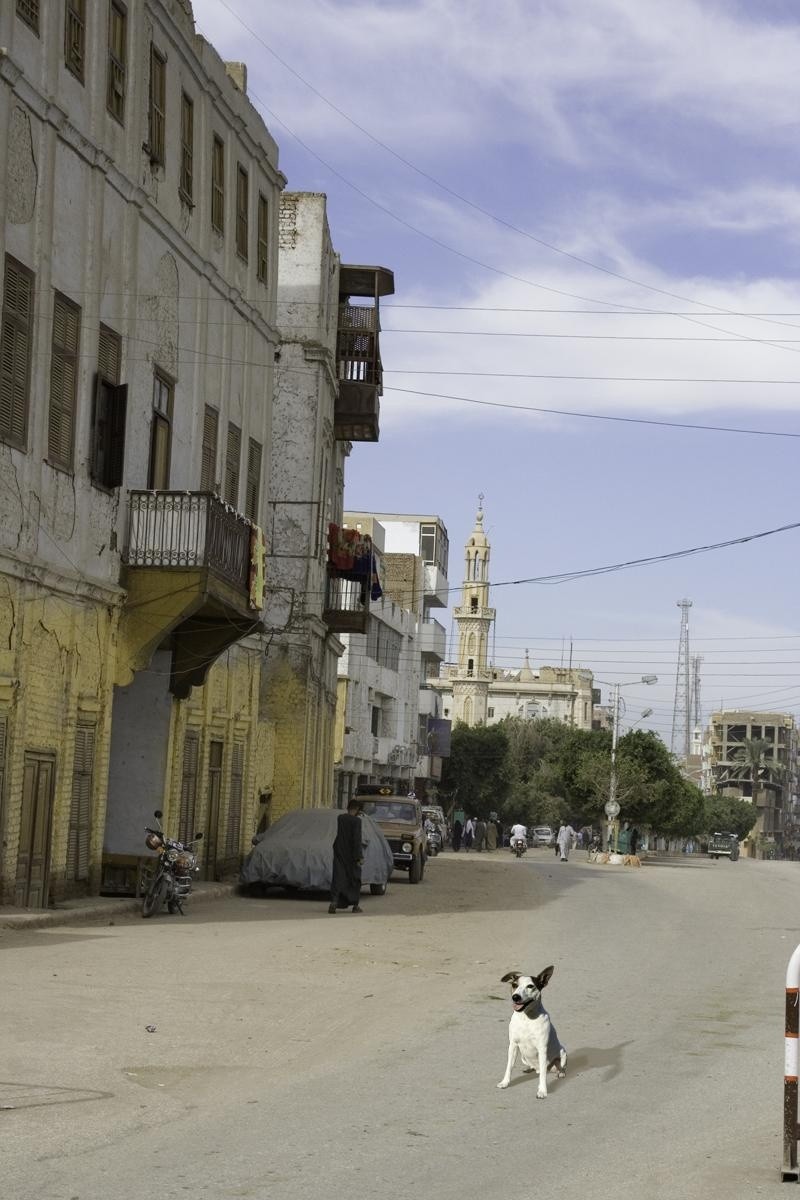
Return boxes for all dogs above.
[495,965,568,1099]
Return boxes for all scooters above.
[426,824,443,858]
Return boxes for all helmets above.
[146,833,163,850]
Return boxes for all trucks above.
[420,806,448,843]
[709,832,739,861]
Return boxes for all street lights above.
[575,671,658,865]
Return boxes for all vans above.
[532,828,553,849]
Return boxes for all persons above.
[453,817,503,853]
[554,819,576,861]
[582,828,589,849]
[510,824,527,854]
[328,799,364,913]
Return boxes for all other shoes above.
[328,904,337,914]
[352,907,363,913]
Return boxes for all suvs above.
[347,796,427,884]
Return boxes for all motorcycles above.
[510,833,530,859]
[140,809,203,919]
